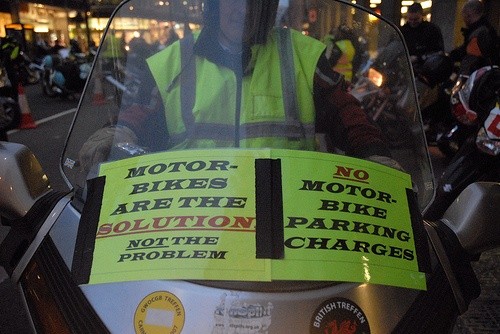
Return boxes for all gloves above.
[368,155,404,172]
[80,125,138,173]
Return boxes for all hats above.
[408,3,422,12]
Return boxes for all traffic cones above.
[16,82,38,128]
[91,73,109,105]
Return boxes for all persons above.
[0,28,179,99]
[397,2,446,65]
[448,0,500,69]
[79,0,420,193]
[320,23,367,84]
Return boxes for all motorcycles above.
[1,0,500,333]
